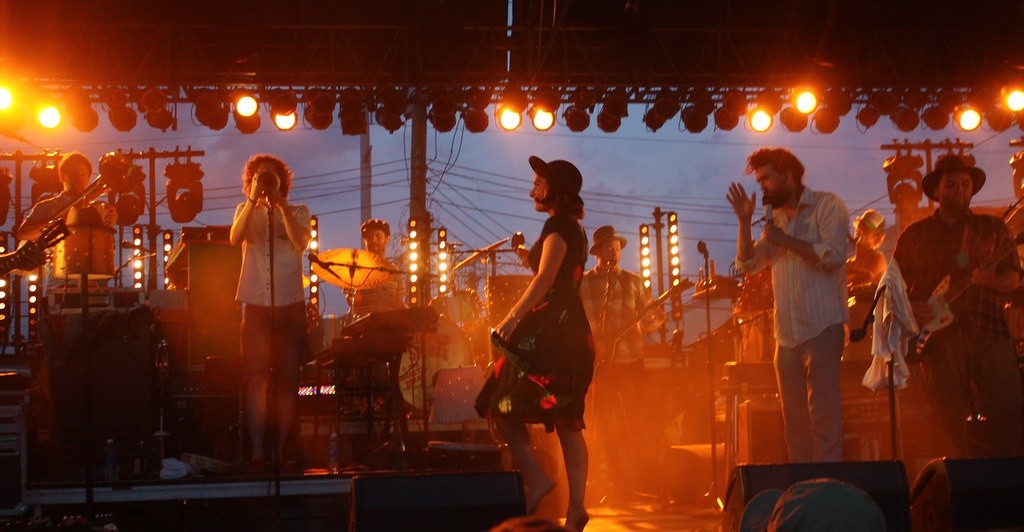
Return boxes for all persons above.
[18,154,118,287]
[576,226,666,505]
[725,144,851,463]
[846,210,889,285]
[474,155,596,531]
[890,153,1023,461]
[229,153,311,473]
[342,219,407,316]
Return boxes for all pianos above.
[338,304,440,471]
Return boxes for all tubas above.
[44,152,140,225]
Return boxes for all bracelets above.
[508,309,518,324]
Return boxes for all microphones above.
[762,195,774,227]
[697,241,709,255]
[308,253,341,280]
[121,240,152,253]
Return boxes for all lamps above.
[0,79,1024,135]
[428,364,488,442]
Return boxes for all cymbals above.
[448,234,510,275]
[310,247,392,291]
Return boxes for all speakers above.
[720,460,909,532]
[909,455,1024,532]
[346,471,527,532]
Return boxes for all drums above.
[434,289,483,330]
[395,313,476,415]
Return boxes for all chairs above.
[80,303,169,481]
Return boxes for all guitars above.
[903,231,1023,366]
[0,218,71,277]
[592,277,694,367]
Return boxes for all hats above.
[740,478,886,532]
[360,219,390,239]
[589,225,628,255]
[489,516,566,532]
[853,209,886,234]
[528,155,585,207]
[922,155,987,202]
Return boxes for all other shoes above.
[524,473,555,515]
[563,509,590,532]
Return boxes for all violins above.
[490,327,579,419]
